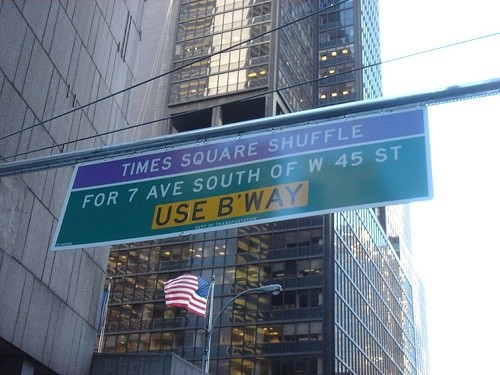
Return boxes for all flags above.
[162,273,211,318]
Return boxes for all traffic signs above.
[50,105,434,250]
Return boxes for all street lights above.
[203,275,282,375]
